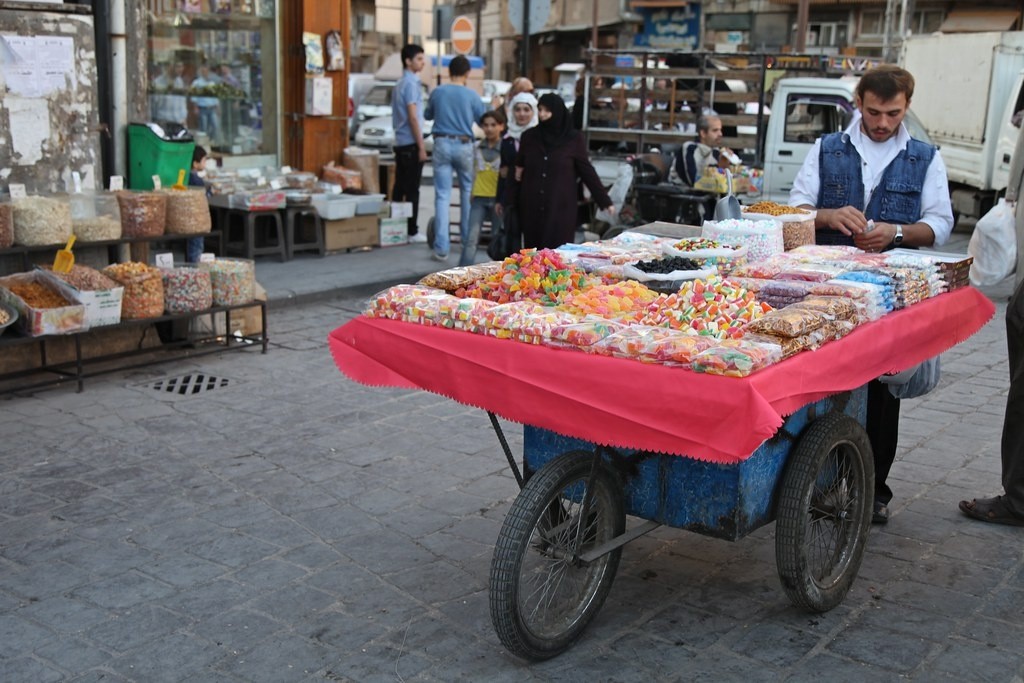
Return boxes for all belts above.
[431,132,470,142]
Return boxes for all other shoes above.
[871,501,890,523]
[844,496,859,519]
[429,250,451,262]
[408,230,427,243]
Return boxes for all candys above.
[103,258,254,319]
[363,219,950,378]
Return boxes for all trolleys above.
[483,369,876,664]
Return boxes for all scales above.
[624,221,702,240]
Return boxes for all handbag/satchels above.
[469,169,500,198]
[487,209,513,262]
[965,198,1019,303]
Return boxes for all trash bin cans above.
[129,119,195,190]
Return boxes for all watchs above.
[894,224,903,246]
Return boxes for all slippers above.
[958,495,1024,525]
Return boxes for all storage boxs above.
[213,164,413,249]
[0,268,85,337]
[35,264,125,327]
[191,285,268,344]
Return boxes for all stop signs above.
[450,17,477,54]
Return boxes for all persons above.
[515,94,615,250]
[573,75,670,155]
[459,110,510,265]
[152,59,240,138]
[390,45,429,242]
[669,115,733,222]
[185,146,221,263]
[788,66,954,524]
[424,56,504,261]
[961,112,1024,526]
[492,79,540,258]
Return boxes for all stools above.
[223,209,286,262]
[266,207,325,258]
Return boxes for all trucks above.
[347,53,485,151]
[890,31,1024,216]
[574,78,932,226]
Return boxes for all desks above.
[0,299,268,394]
[0,229,222,272]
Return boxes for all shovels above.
[53,234,77,274]
[174,170,187,190]
[715,168,742,220]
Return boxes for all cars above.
[356,80,509,165]
